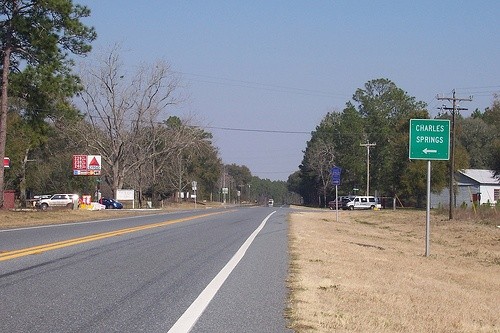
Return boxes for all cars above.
[98,198,124,209]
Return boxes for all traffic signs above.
[409,120,451,161]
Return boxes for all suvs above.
[37,193,81,209]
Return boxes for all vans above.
[329,194,379,210]
